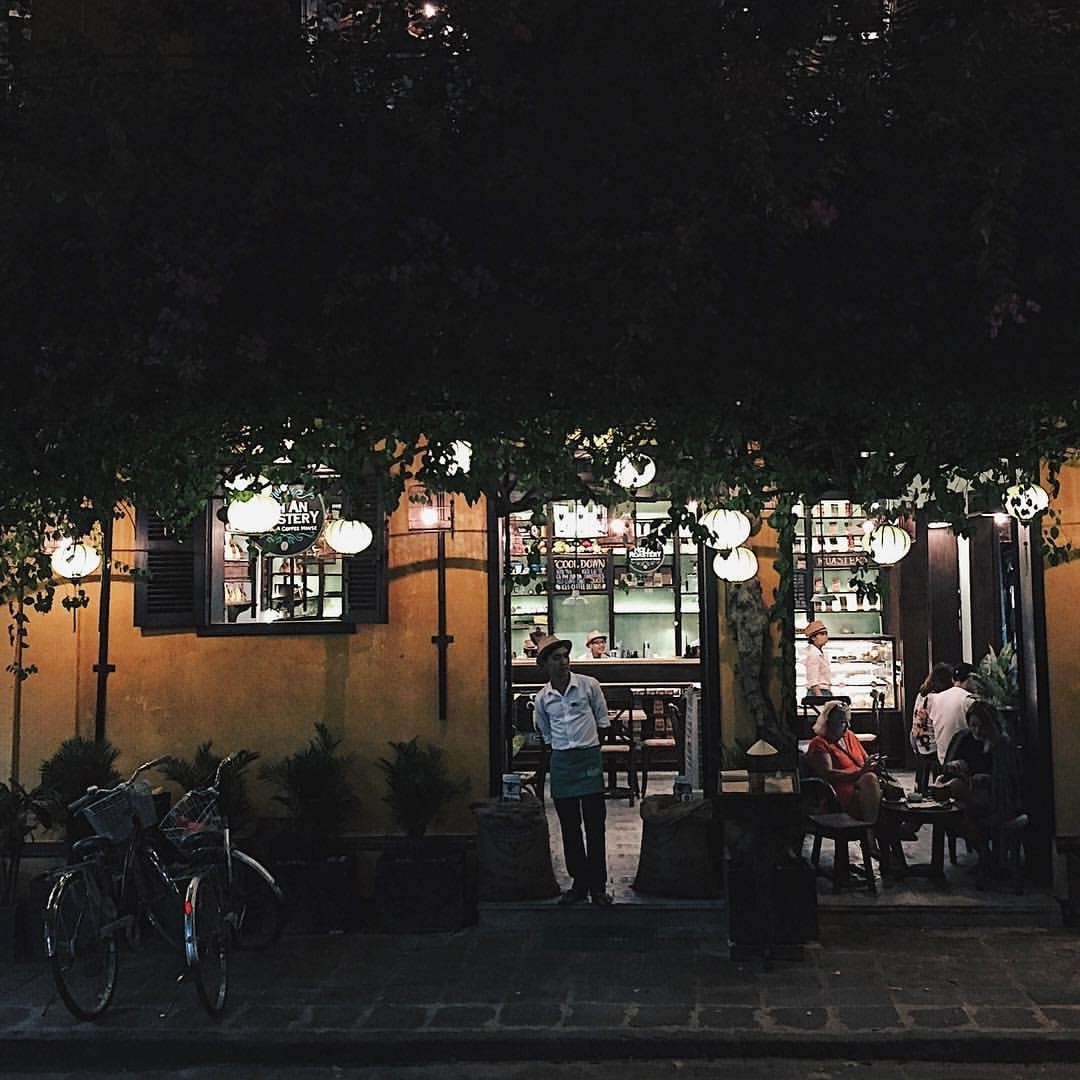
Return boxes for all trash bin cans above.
[721,766,821,963]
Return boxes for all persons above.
[910,662,1024,872]
[534,635,611,905]
[576,630,613,659]
[807,700,902,872]
[804,623,833,697]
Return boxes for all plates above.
[882,797,907,803]
[923,798,956,803]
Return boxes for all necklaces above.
[840,739,845,749]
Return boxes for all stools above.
[797,811,878,891]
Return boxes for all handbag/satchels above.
[910,698,936,756]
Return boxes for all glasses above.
[828,702,847,716]
[592,641,604,644]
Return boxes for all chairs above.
[799,692,1029,882]
[508,698,691,799]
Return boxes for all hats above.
[807,621,828,637]
[536,636,571,655]
[586,630,607,647]
[953,662,976,679]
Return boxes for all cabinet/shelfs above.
[793,500,891,614]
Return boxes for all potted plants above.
[0,714,478,964]
[974,642,1023,733]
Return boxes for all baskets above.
[159,790,221,847]
[83,780,159,840]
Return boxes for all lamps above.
[613,453,655,486]
[438,441,477,475]
[713,546,758,584]
[51,530,102,577]
[324,518,375,556]
[999,481,1050,522]
[696,506,749,552]
[227,491,280,532]
[863,521,912,566]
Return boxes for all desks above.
[598,708,649,796]
[875,797,962,885]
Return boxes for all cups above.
[644,571,663,587]
[794,503,877,611]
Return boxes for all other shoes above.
[863,847,883,860]
[593,890,611,904]
[567,886,588,900]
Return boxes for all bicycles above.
[41,753,199,1024]
[156,747,290,1020]
